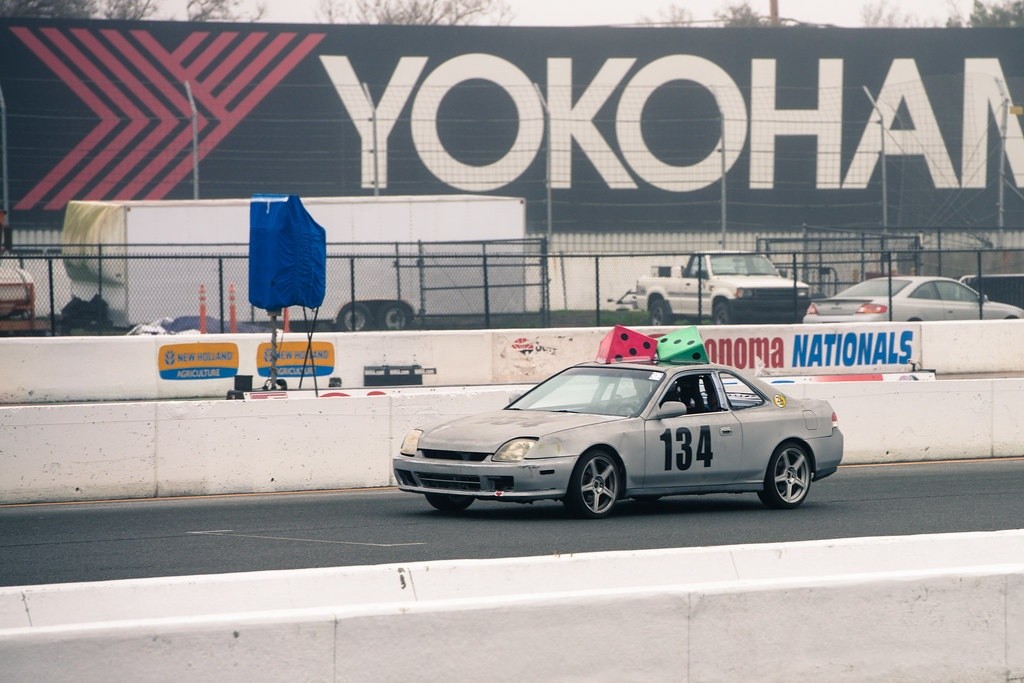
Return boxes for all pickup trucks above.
[635,248,812,326]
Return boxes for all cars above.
[393,359,845,520]
[802,274,1024,324]
